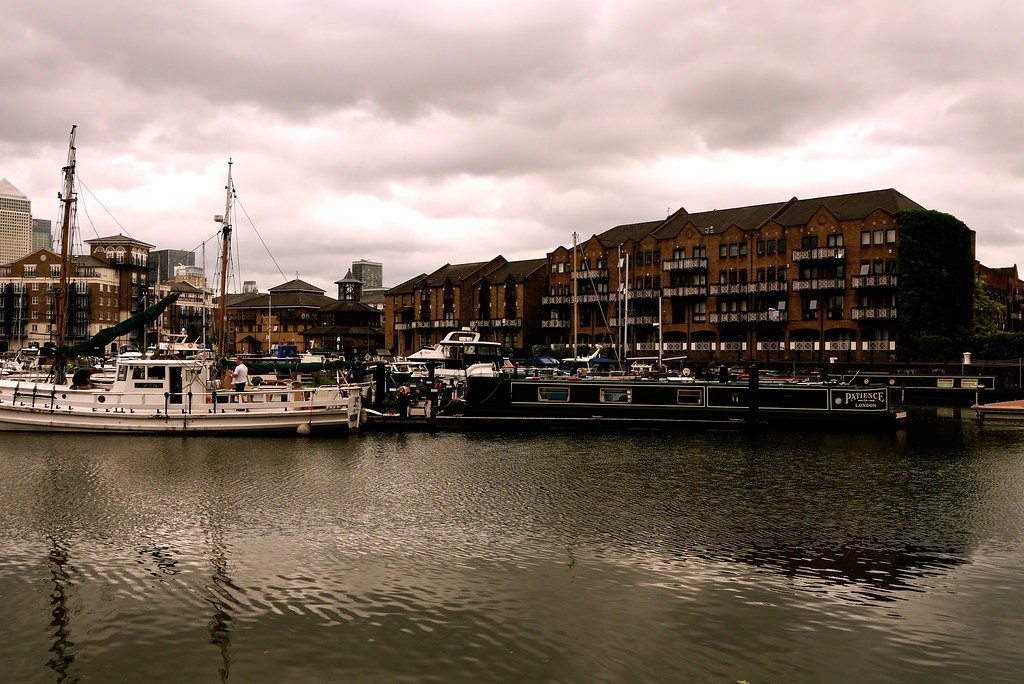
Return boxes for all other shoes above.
[70,385,78,390]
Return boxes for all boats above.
[407,328,501,377]
[0,122,364,434]
[149,332,199,358]
[433,231,998,432]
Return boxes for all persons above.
[230,357,248,392]
[70,367,97,389]
